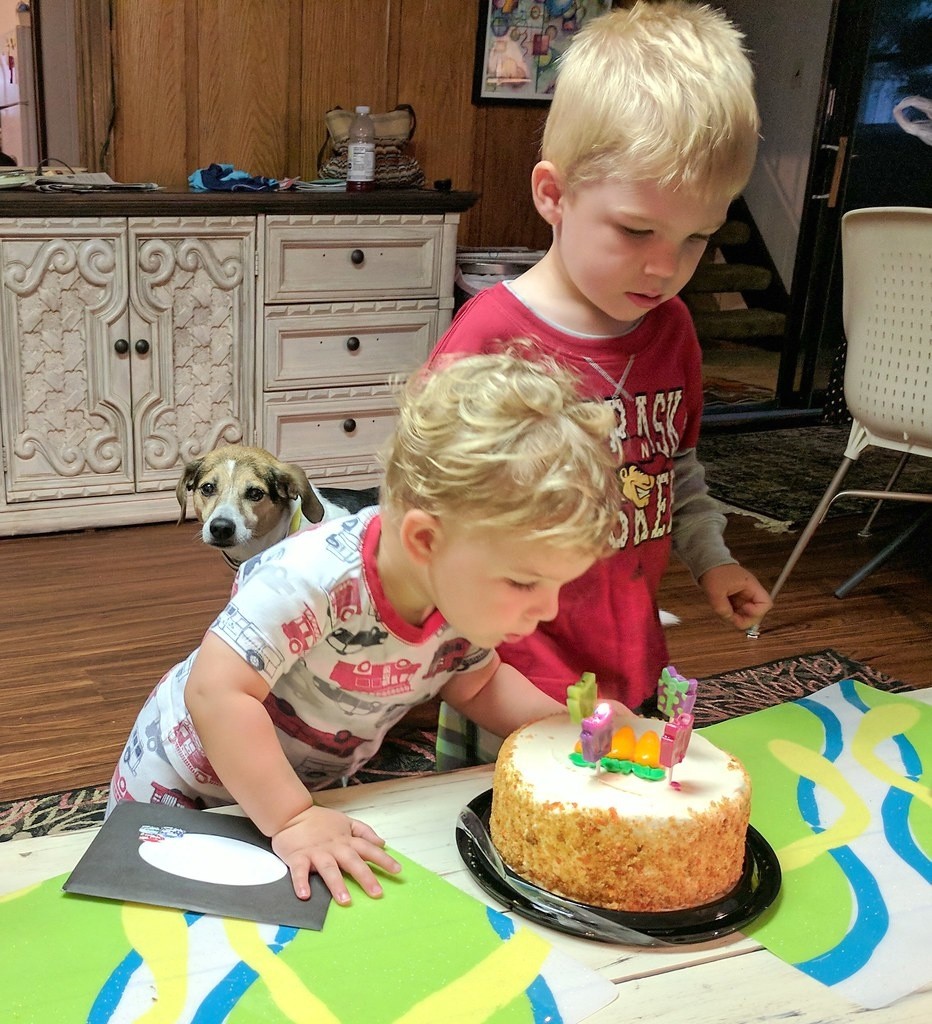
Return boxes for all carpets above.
[1,646,919,845]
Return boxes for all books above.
[270,175,348,192]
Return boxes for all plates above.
[457,787,781,948]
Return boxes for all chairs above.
[754,206,932,631]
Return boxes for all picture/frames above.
[470,0,623,110]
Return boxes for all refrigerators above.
[0,26,41,171]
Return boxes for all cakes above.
[490,665,753,914]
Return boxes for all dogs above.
[177,445,380,575]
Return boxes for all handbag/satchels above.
[314,100,424,189]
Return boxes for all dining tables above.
[1,687,932,1024]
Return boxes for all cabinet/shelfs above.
[1,218,256,534]
[261,215,461,490]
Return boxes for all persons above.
[106,333,628,907]
[412,1,776,778]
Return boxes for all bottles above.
[345,106,376,193]
